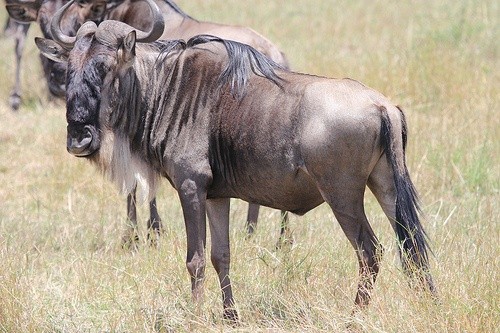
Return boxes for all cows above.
[1,1,295,251]
[34,0,439,326]
